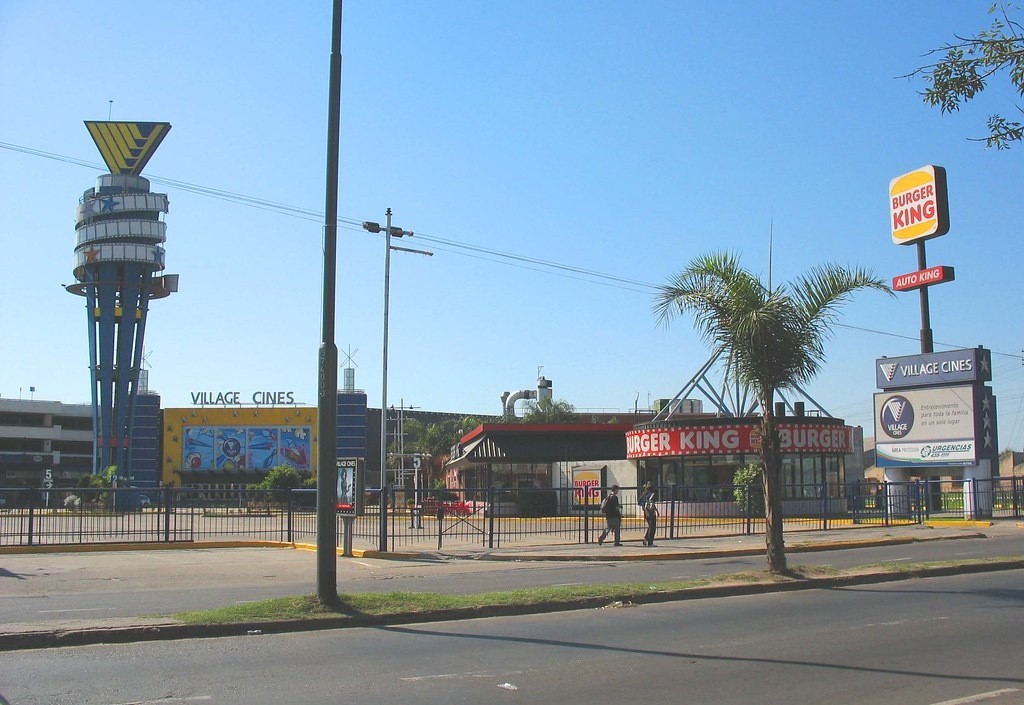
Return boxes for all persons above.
[637,481,660,547]
[598,484,623,546]
[341,467,351,504]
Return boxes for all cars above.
[136,494,151,508]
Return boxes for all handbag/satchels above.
[645,493,655,510]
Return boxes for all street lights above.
[362,208,414,551]
[30,386,35,399]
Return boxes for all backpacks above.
[602,494,613,512]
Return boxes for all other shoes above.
[643,538,646,547]
[614,543,622,546]
[598,538,602,545]
[648,544,657,547]
